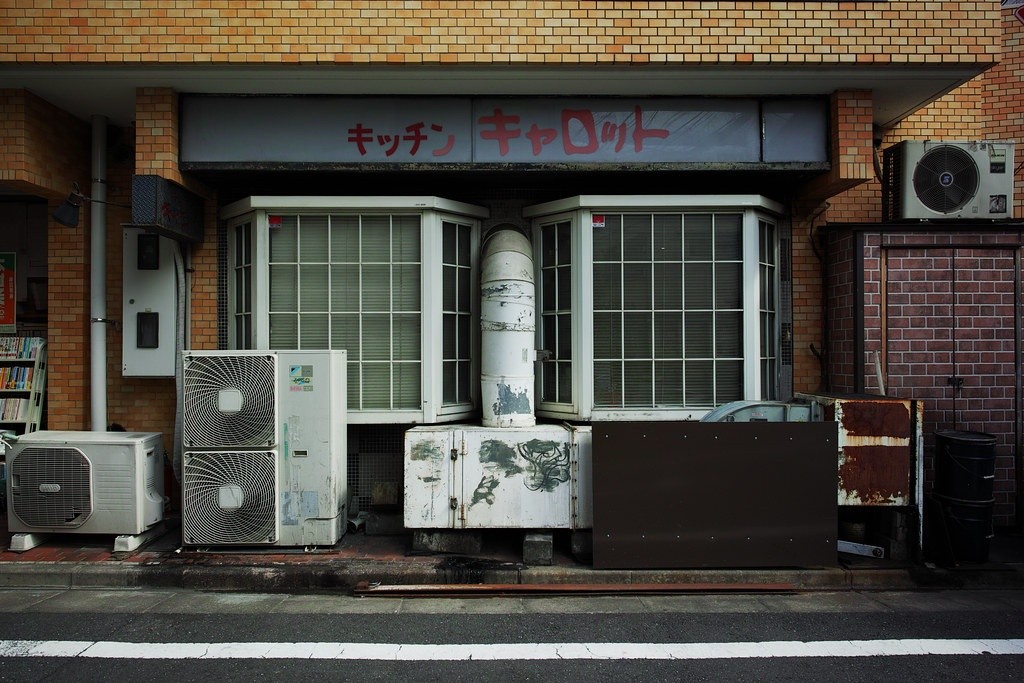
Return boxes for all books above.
[0,337,47,422]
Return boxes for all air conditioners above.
[180,348,348,544]
[5,430,165,533]
[883,140,1014,218]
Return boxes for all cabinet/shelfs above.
[0,336,47,463]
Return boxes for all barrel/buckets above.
[933,430,998,502]
[932,492,995,566]
